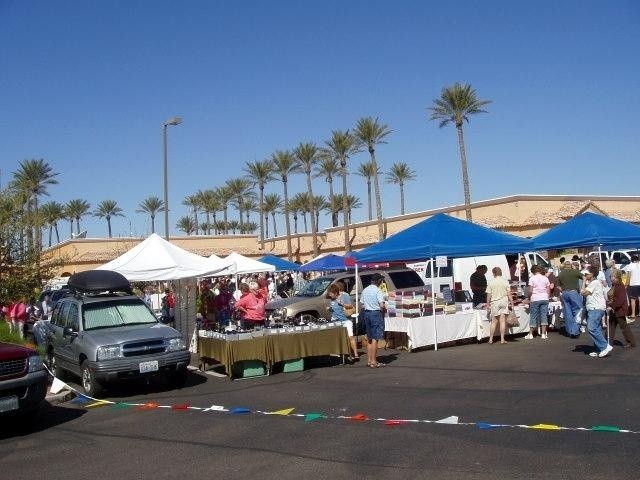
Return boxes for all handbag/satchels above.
[621,271,630,286]
[343,304,356,317]
[506,307,521,327]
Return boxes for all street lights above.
[164,116,180,243]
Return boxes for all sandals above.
[636,315,640,317]
[370,363,385,368]
[354,355,360,361]
[488,341,493,344]
[367,362,370,366]
[627,316,635,319]
[500,341,507,344]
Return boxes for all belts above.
[563,289,576,291]
[362,310,381,312]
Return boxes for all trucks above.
[580,249,640,280]
[509,252,557,298]
[424,255,512,300]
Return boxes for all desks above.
[464,300,564,345]
[384,310,477,353]
[198,336,271,381]
[268,326,351,376]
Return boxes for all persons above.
[359,274,386,367]
[485,267,514,344]
[580,266,613,358]
[623,256,640,319]
[545,267,557,297]
[606,270,636,348]
[525,266,550,339]
[142,287,175,327]
[558,261,586,337]
[571,262,586,294]
[2,300,26,339]
[592,259,607,328]
[326,284,359,360]
[605,258,616,287]
[470,265,487,303]
[200,275,236,327]
[240,274,266,289]
[235,281,267,328]
[265,272,309,302]
[336,282,350,297]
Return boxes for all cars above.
[39,288,69,322]
[0,341,49,416]
[46,269,192,397]
[263,265,427,329]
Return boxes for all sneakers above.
[589,352,597,357]
[603,324,607,327]
[582,321,588,325]
[541,334,547,339]
[525,334,533,339]
[599,345,613,357]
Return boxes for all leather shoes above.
[622,344,635,348]
[611,344,614,346]
[574,335,578,338]
[570,334,574,338]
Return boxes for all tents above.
[208,254,223,262]
[257,255,302,299]
[349,213,534,350]
[224,252,275,299]
[301,251,330,266]
[95,234,231,314]
[532,211,640,274]
[301,254,349,276]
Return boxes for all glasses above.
[585,273,592,276]
[165,292,170,293]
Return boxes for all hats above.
[165,288,170,293]
[371,273,385,281]
[267,278,272,281]
